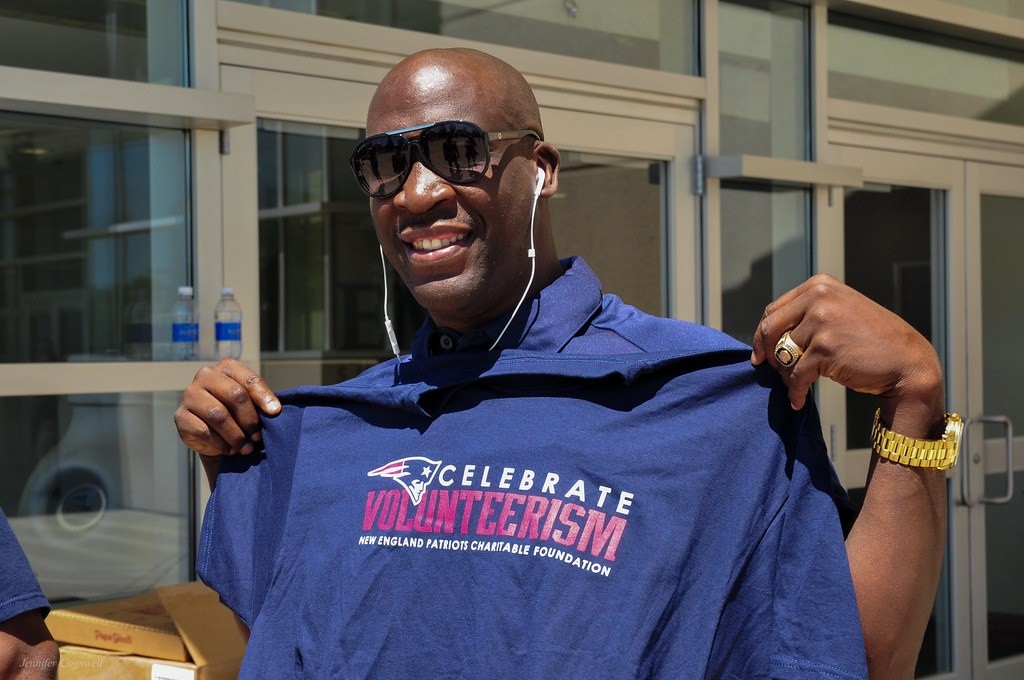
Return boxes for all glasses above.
[349,119,540,200]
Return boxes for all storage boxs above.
[45,579,252,680]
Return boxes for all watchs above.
[870,408,964,471]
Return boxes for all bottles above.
[171,286,199,360]
[214,288,242,361]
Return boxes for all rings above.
[774,331,804,367]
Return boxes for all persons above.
[174,47,964,680]
[0,509,61,680]
